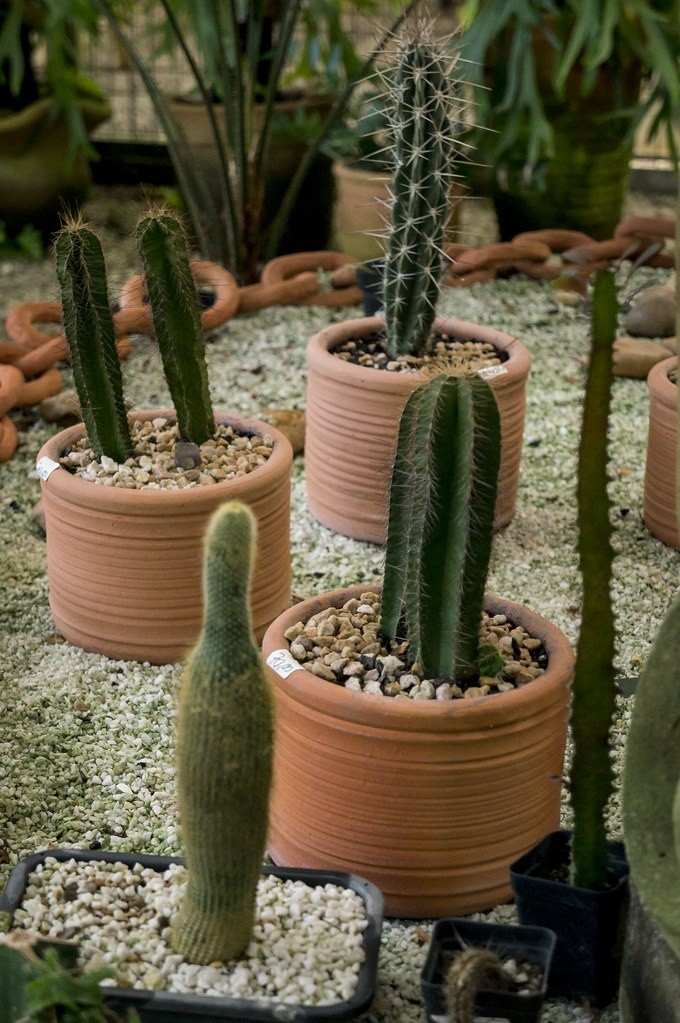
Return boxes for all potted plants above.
[0,499,385,1023]
[37,205,294,664]
[418,271,680,1023]
[261,364,577,923]
[300,5,527,542]
[0,0,680,276]
